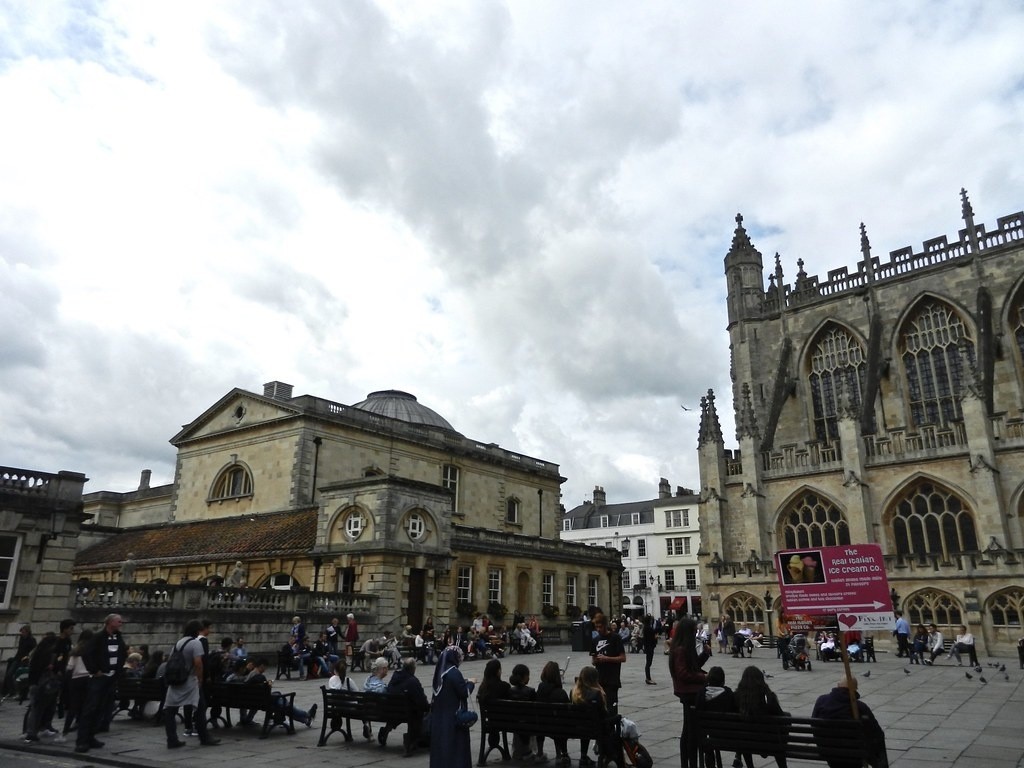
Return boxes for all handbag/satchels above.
[716,629,722,642]
[616,718,653,768]
[457,687,478,729]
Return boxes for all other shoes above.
[955,662,963,667]
[363,724,412,757]
[306,703,317,728]
[944,657,951,660]
[21,724,105,752]
[244,722,261,728]
[167,728,222,749]
[925,659,933,665]
[561,756,570,768]
[523,752,534,760]
[579,757,595,768]
[733,759,743,768]
[535,753,548,763]
[646,678,656,685]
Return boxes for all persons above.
[589,612,627,755]
[669,618,713,768]
[69,613,128,753]
[115,552,137,605]
[206,560,250,608]
[0,608,1024,768]
[25,635,66,741]
[429,646,478,768]
[810,673,890,768]
[162,618,223,749]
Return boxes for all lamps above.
[34,507,67,565]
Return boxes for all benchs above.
[740,634,785,658]
[350,645,402,672]
[110,674,185,728]
[509,630,545,654]
[315,685,430,755]
[690,704,882,768]
[200,680,296,737]
[274,650,300,679]
[907,636,977,667]
[831,635,877,663]
[474,696,623,768]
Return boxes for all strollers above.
[783,634,812,671]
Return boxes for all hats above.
[347,613,354,619]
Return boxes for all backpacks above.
[164,637,195,685]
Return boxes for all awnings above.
[668,597,687,610]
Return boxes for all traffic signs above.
[776,544,895,631]
